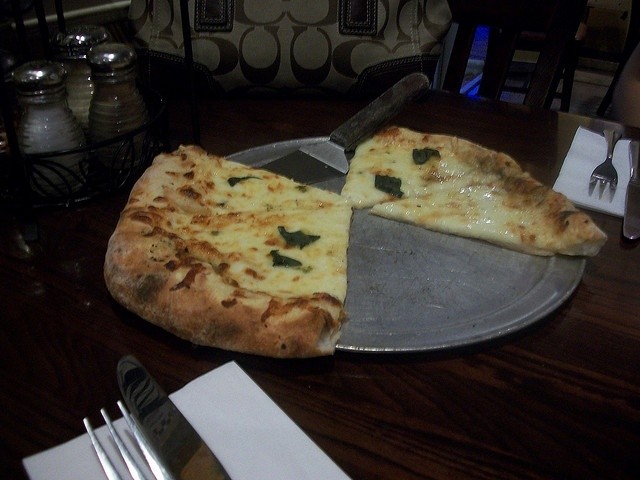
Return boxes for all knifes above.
[622,138,639,238]
[115,353,232,479]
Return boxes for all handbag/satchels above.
[127,2,454,99]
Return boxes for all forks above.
[587,121,627,201]
[81,401,171,479]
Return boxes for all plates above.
[168,138,586,352]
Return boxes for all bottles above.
[87,42,156,202]
[9,61,91,223]
[56,26,107,130]
[90,58,94,59]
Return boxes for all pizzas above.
[340,125,609,258]
[102,143,352,358]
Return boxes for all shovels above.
[256,71,430,186]
